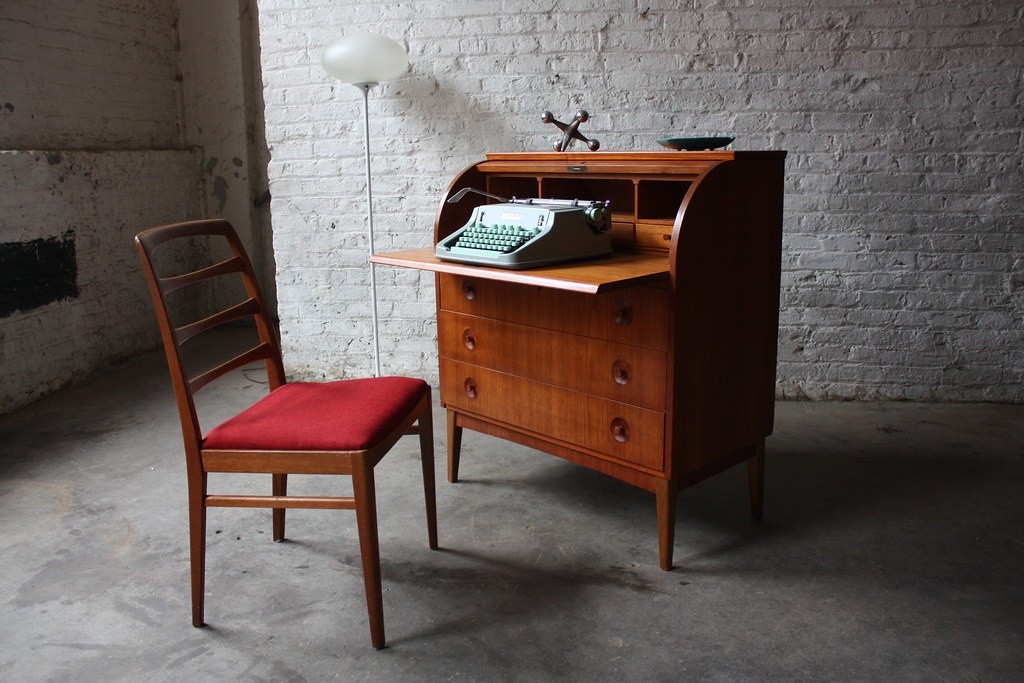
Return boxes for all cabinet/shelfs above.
[366,146,788,571]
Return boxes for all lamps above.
[320,36,412,378]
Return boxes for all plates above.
[656,136,735,151]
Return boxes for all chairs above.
[133,218,439,652]
